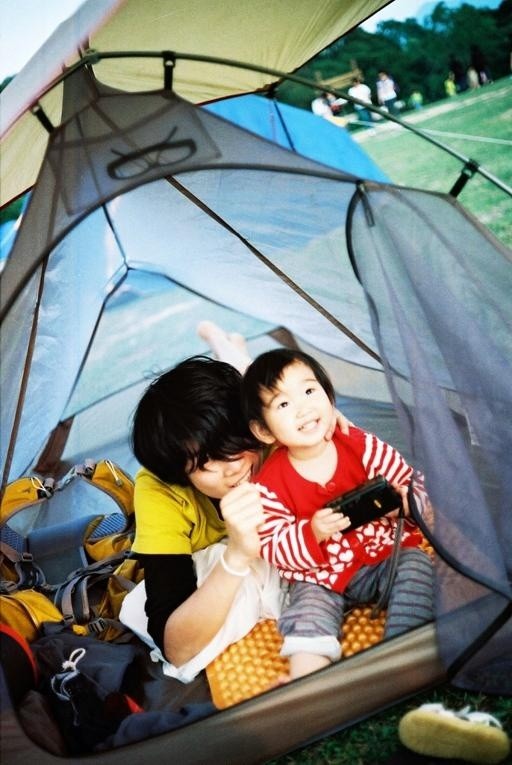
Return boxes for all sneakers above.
[399,702,510,763]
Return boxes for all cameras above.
[325,474,403,534]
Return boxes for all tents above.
[0,0,512,765]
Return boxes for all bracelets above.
[219,554,251,577]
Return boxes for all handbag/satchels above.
[2,460,135,641]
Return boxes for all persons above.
[241,346,440,684]
[127,320,255,668]
[313,65,488,131]
